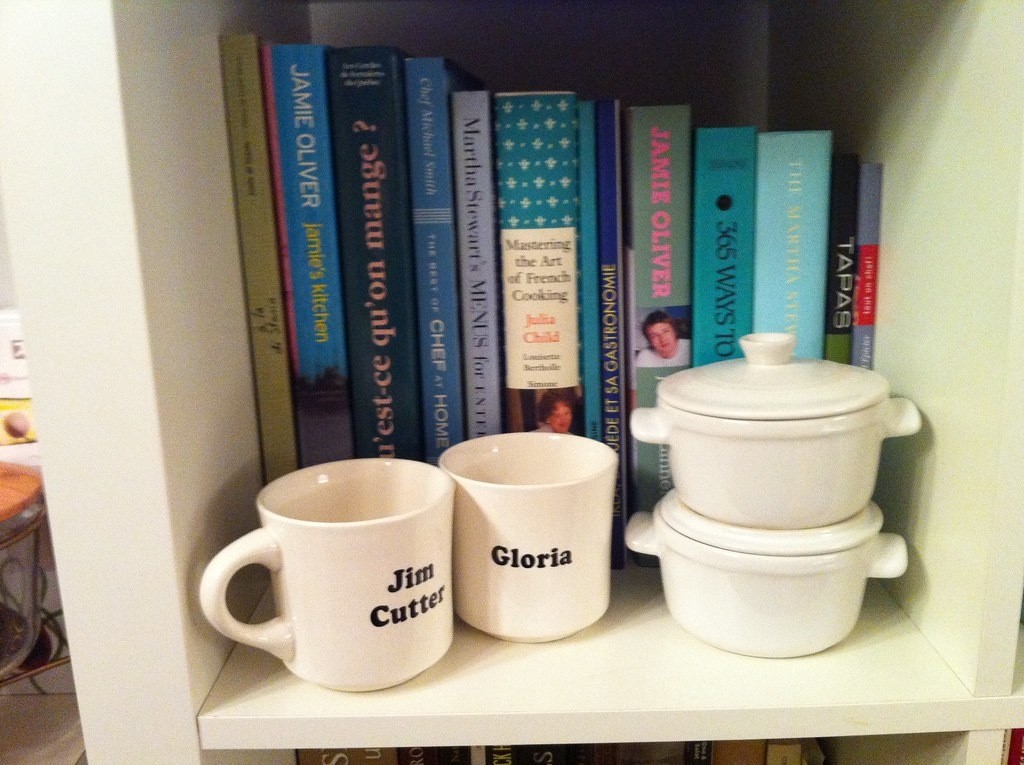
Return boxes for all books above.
[221,33,883,569]
[298,739,805,765]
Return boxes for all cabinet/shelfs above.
[2,0,1024,765]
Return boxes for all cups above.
[199,457,457,693]
[438,432,619,644]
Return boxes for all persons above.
[534,388,578,434]
[637,311,691,366]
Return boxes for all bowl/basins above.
[630,332,922,532]
[623,489,910,660]
